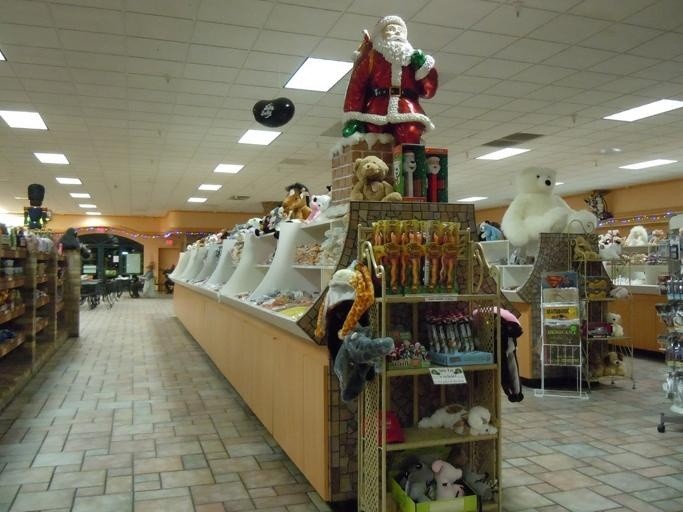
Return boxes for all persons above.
[139,266,155,298]
[341,16,438,144]
[162,264,175,293]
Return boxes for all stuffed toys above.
[498,165,598,247]
[394,402,498,502]
[473,305,527,404]
[479,221,504,241]
[349,155,403,201]
[331,325,395,403]
[574,225,668,379]
[313,259,376,367]
[186,182,350,267]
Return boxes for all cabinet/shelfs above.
[169,202,479,503]
[475,236,676,397]
[355,220,502,512]
[2,238,66,417]
